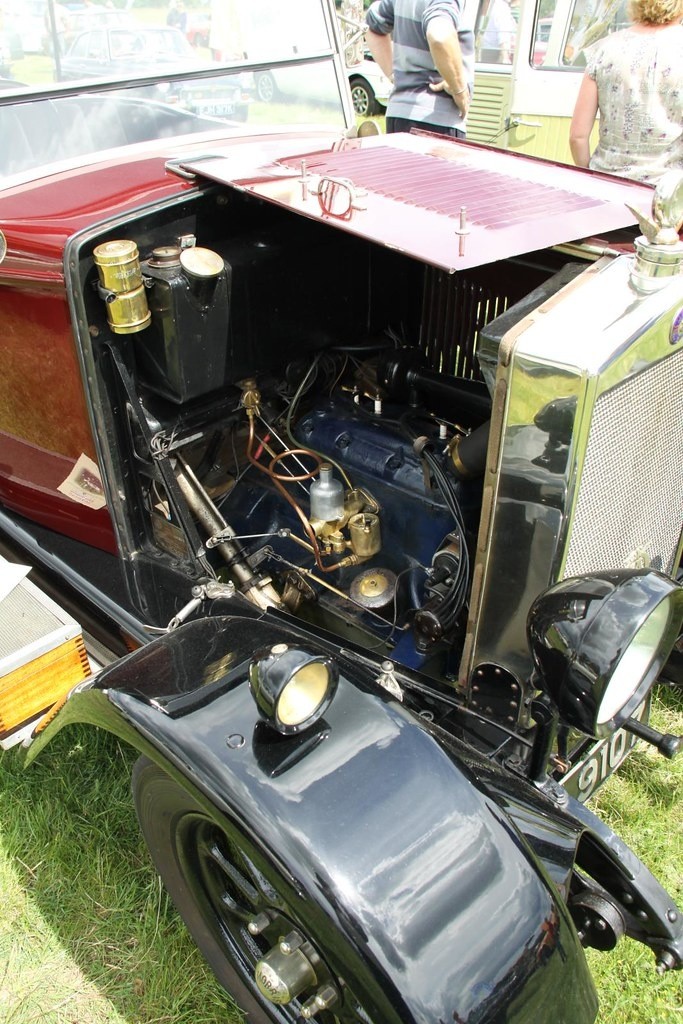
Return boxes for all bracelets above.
[454,81,468,94]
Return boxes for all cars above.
[56,26,249,125]
[253,39,394,119]
[507,17,552,66]
[1,0,683,1024]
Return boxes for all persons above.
[166,2,188,33]
[480,0,517,64]
[365,0,476,139]
[569,0,683,187]
